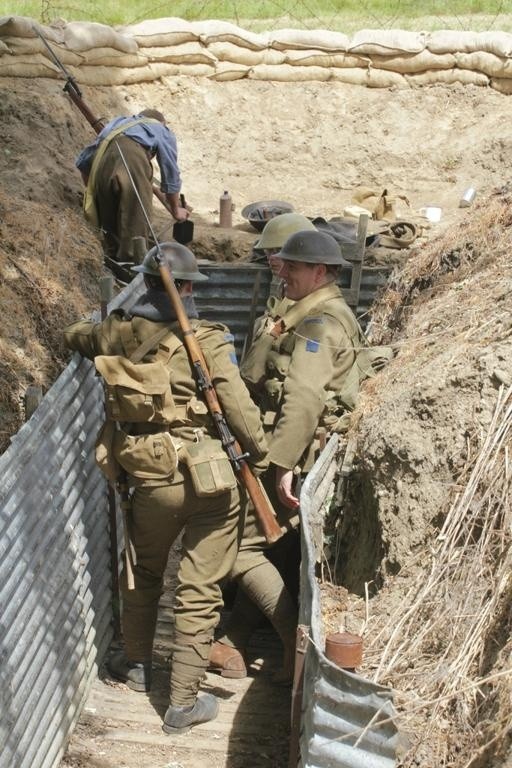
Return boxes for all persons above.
[75,109,190,266]
[62,242,269,735]
[203,213,361,685]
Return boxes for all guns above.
[115,142,283,544]
[30,27,193,222]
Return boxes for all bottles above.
[185,426,237,497]
[220,191,232,227]
[459,187,477,208]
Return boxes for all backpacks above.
[94,355,176,425]
[323,298,395,412]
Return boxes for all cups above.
[420,207,441,224]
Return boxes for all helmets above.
[130,242,209,281]
[241,200,343,265]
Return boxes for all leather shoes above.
[270,673,293,688]
[162,694,220,734]
[205,643,247,678]
[107,652,152,692]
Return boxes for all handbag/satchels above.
[83,178,97,221]
[241,328,276,384]
[95,421,120,481]
